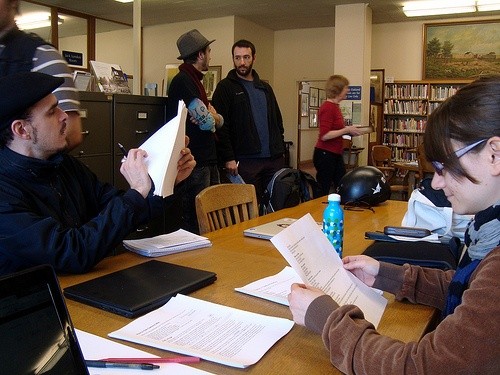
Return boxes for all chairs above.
[195,184,258,236]
[371,144,435,200]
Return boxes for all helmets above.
[337,166,391,204]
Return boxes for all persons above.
[167,30,224,235]
[210,39,285,201]
[0,0,83,154]
[0,72,197,274]
[313,75,361,194]
[287,74,499,375]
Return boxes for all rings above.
[121,159,125,162]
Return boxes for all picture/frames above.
[370,68,385,103]
[201,65,222,102]
[421,18,500,81]
[383,80,474,164]
[301,87,327,128]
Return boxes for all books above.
[383,84,460,161]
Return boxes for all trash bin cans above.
[284,142,293,168]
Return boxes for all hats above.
[0,72,64,113]
[176,29,215,58]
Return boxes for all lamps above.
[401,0,500,18]
[16,16,63,30]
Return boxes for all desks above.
[58,193,437,375]
[393,160,419,202]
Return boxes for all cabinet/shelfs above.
[75,93,177,188]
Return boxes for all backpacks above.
[257,166,304,216]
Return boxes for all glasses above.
[432,137,491,176]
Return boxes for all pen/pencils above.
[85,360,159,370]
[99,356,201,364]
[117,142,128,157]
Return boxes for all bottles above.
[322,193,344,260]
